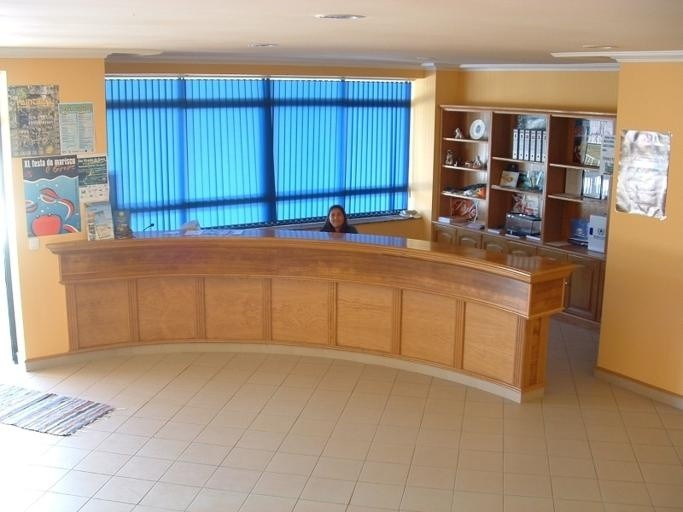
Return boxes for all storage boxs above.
[584,215,606,254]
[504,211,542,237]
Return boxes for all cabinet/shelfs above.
[478,233,534,260]
[541,111,615,260]
[538,245,605,323]
[430,222,481,249]
[430,105,491,235]
[489,108,547,243]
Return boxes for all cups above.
[398,209,422,219]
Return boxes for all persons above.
[320,204,358,234]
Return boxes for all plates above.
[468,118,485,140]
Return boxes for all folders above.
[512,130,547,163]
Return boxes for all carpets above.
[0,385,115,436]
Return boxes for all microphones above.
[143,224,154,231]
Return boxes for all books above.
[437,215,466,224]
[84,200,114,241]
[510,128,548,163]
